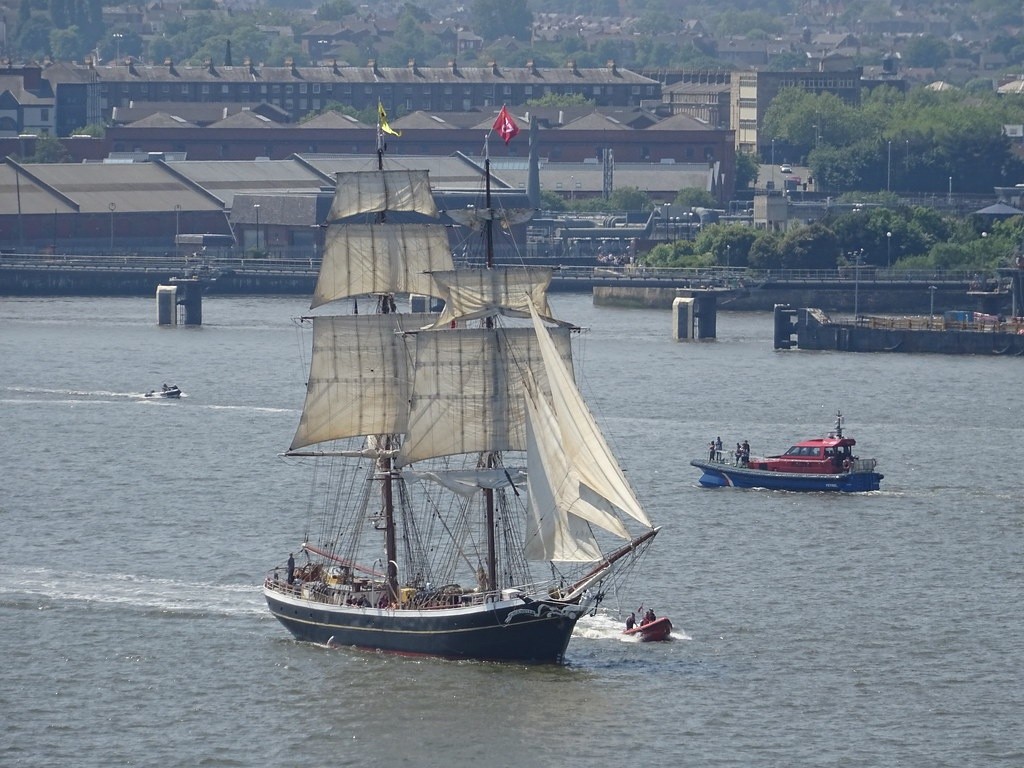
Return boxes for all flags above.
[492,104,521,146]
[377,102,402,138]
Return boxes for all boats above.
[690,409,884,493]
[145,385,181,398]
[622,617,673,643]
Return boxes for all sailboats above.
[259,95,662,668]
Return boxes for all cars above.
[790,175,801,185]
[781,163,792,174]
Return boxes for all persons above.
[714,436,723,462]
[354,578,372,592]
[626,613,638,631]
[733,441,750,468]
[640,608,656,627]
[287,552,294,585]
[346,593,389,609]
[707,440,715,463]
[162,383,171,391]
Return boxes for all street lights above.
[254,204,260,258]
[848,248,864,317]
[669,216,680,245]
[317,40,327,65]
[683,212,694,241]
[772,139,775,179]
[949,176,952,200]
[811,110,824,150]
[927,285,938,316]
[663,202,671,240]
[174,204,181,258]
[887,141,892,192]
[113,34,123,67]
[886,232,892,268]
[109,203,116,249]
[905,140,909,158]
